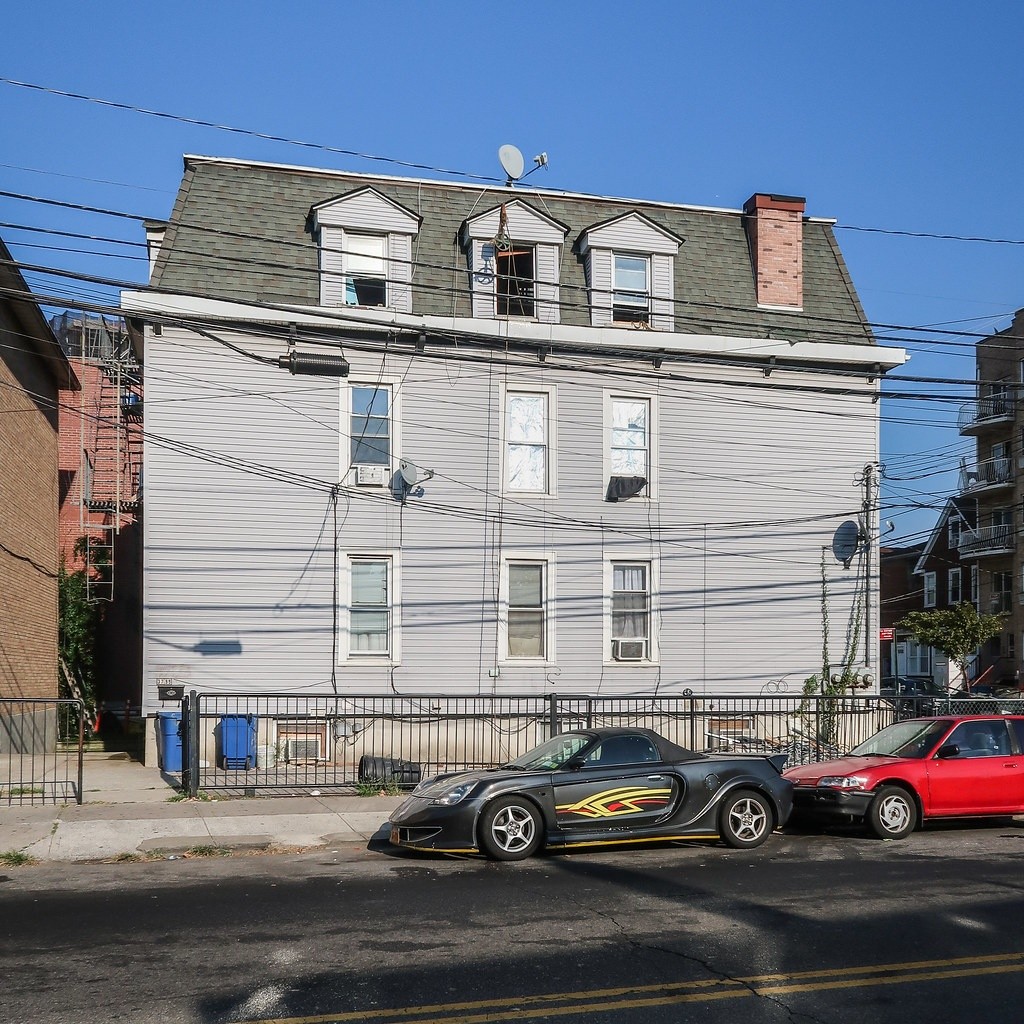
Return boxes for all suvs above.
[880,674,949,720]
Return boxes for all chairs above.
[959,732,993,756]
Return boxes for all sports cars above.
[366,727,798,861]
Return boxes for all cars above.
[950,684,1024,714]
[778,708,1024,840]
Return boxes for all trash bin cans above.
[213,715,259,770]
[154,711,191,772]
